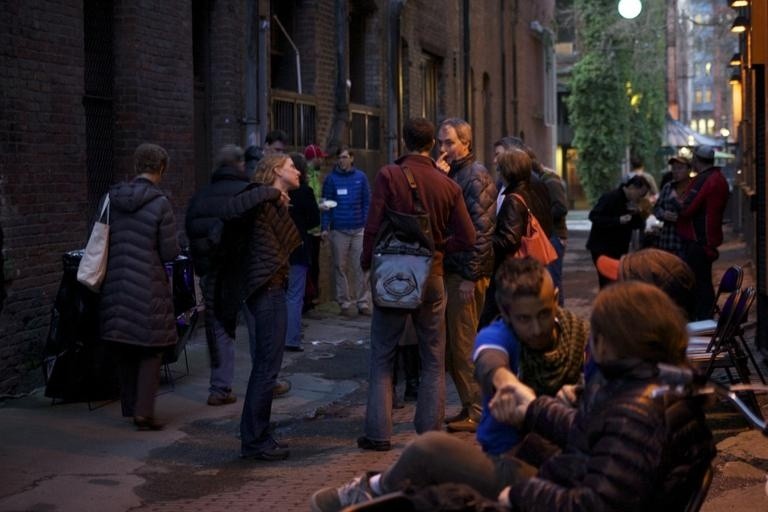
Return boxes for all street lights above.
[173,249,197,316]
[510,193,559,265]
[76,193,111,293]
[370,165,435,309]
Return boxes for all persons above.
[184,130,373,461]
[587,145,728,323]
[356,118,569,451]
[471,257,589,461]
[312,283,718,511]
[98,143,187,432]
[617,252,689,310]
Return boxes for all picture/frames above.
[49,244,193,346]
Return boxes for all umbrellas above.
[444,406,468,423]
[276,440,288,448]
[240,448,290,461]
[357,438,392,452]
[272,381,290,396]
[207,394,237,406]
[447,418,476,432]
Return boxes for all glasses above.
[728,15,748,86]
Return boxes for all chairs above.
[360,308,371,315]
[341,304,358,318]
[311,469,381,512]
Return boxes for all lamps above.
[678,263,763,436]
[155,308,200,390]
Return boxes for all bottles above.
[694,144,714,159]
[305,144,329,160]
[668,156,688,164]
[595,247,695,296]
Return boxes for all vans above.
[134,416,163,430]
